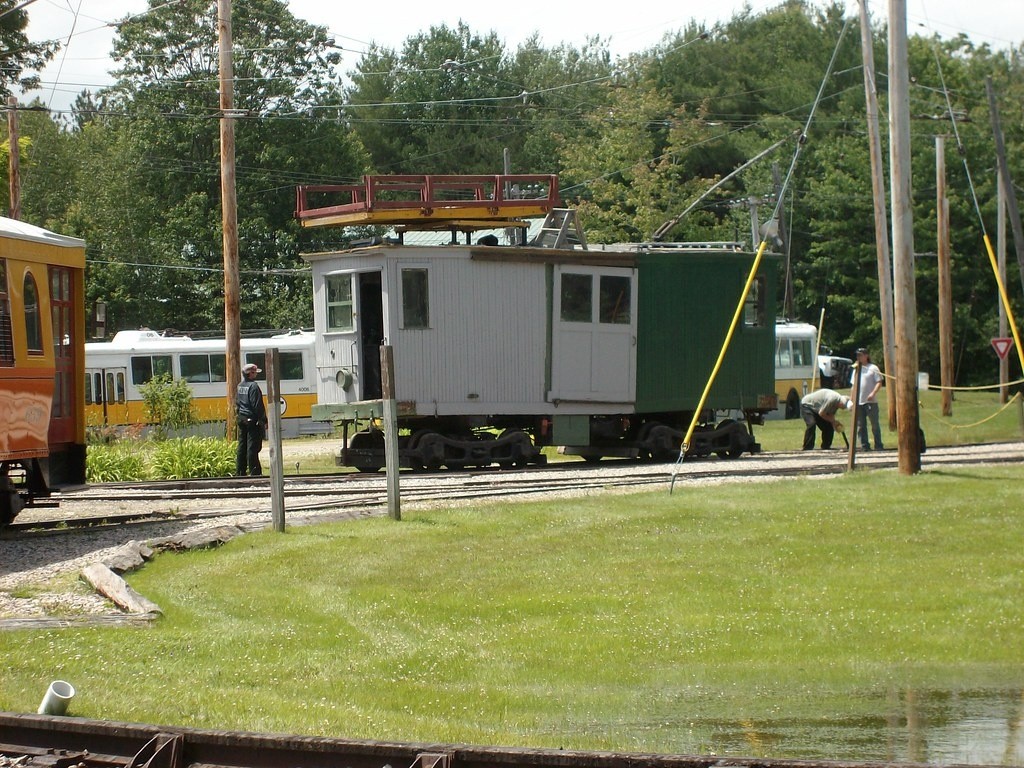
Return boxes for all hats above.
[855,348,868,354]
[243,364,262,374]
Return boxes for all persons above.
[800,388,853,450]
[847,348,884,452]
[235,364,268,475]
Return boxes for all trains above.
[83,321,822,430]
[1,217,87,532]
